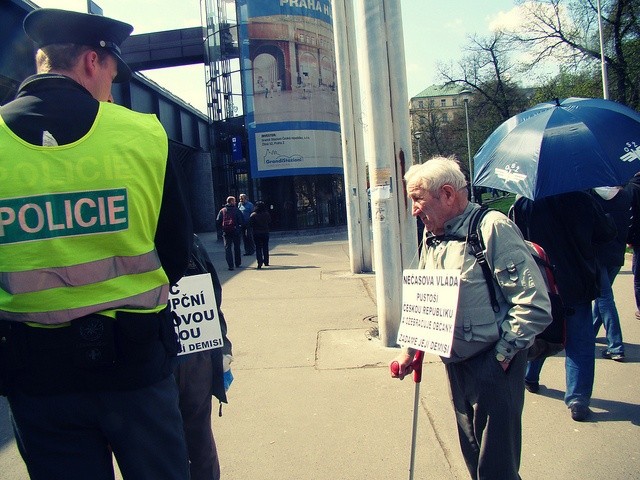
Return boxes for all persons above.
[589,185,626,360]
[238,194,255,256]
[626,172,640,320]
[0,7,190,480]
[216,196,245,271]
[177,235,234,480]
[507,192,617,421]
[248,201,271,269]
[389,154,553,480]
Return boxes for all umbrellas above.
[472,96,640,203]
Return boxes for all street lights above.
[459,89,475,203]
[414,131,423,166]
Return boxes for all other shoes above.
[525,382,540,393]
[602,349,624,360]
[228,266,234,271]
[236,264,240,269]
[571,405,589,421]
[243,253,253,256]
[265,263,269,266]
[257,264,262,269]
[635,311,640,320]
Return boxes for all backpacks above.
[469,205,566,361]
[221,207,236,230]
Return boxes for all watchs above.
[495,351,511,364]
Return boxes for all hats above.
[24,8,133,84]
